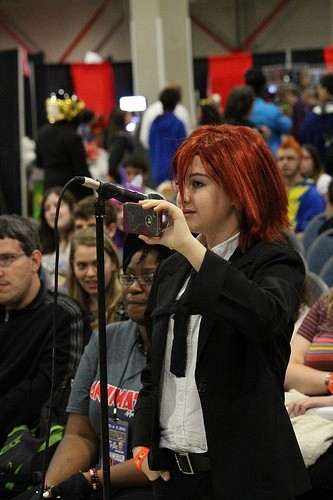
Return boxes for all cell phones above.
[124,202,162,236]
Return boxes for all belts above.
[159,448,211,475]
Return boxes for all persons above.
[0,213,92,500]
[282,287,333,416]
[34,64,333,443]
[128,125,312,500]
[40,244,172,500]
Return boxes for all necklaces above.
[135,327,147,358]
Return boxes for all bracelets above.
[88,468,101,491]
[132,447,151,472]
[323,371,333,395]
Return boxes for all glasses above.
[0,253,25,266]
[118,274,154,285]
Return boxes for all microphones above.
[74,175,164,204]
